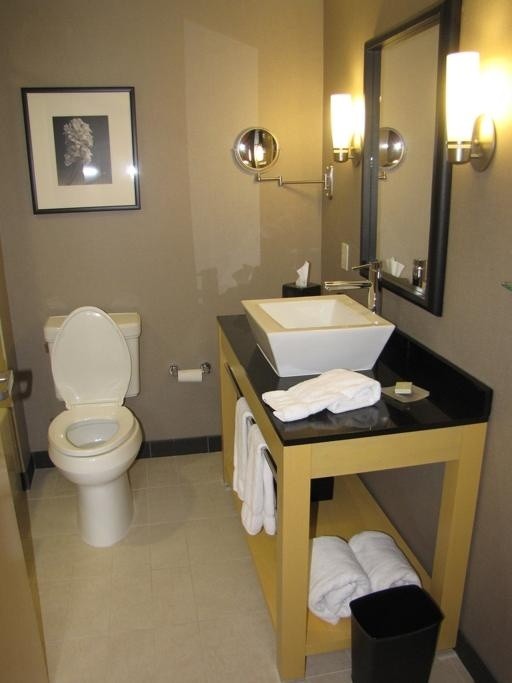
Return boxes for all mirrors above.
[232,126,334,197]
[360,2,463,317]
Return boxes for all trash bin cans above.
[345,581,444,683]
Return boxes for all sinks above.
[241,293,395,377]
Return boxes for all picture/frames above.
[20,86,141,215]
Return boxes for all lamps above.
[330,92,363,167]
[445,51,496,174]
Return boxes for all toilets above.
[49,306,142,546]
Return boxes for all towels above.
[261,369,383,422]
[232,397,253,501]
[240,424,277,536]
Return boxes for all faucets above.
[352,260,382,316]
